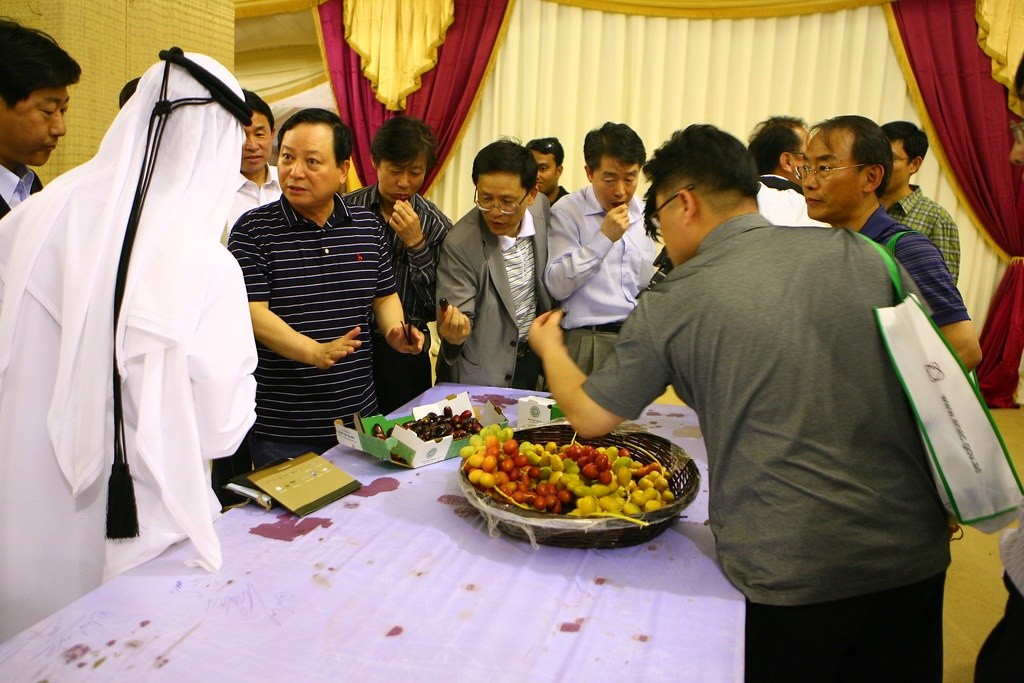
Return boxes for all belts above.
[581,322,624,333]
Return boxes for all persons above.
[745,115,984,375]
[526,137,569,208]
[543,123,661,380]
[435,137,553,391]
[973,500,1024,683]
[0,18,83,220]
[0,46,259,643]
[209,88,424,510]
[528,123,958,683]
[342,113,452,416]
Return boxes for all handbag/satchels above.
[854,231,1024,535]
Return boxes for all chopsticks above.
[401,315,414,344]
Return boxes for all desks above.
[0,383,747,683]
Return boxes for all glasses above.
[649,184,694,229]
[527,139,553,151]
[1009,120,1024,145]
[794,163,864,181]
[474,186,529,215]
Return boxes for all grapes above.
[439,298,449,311]
[458,423,675,521]
[373,406,486,464]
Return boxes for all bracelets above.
[404,234,425,249]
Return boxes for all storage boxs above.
[334,391,509,469]
[517,394,564,427]
